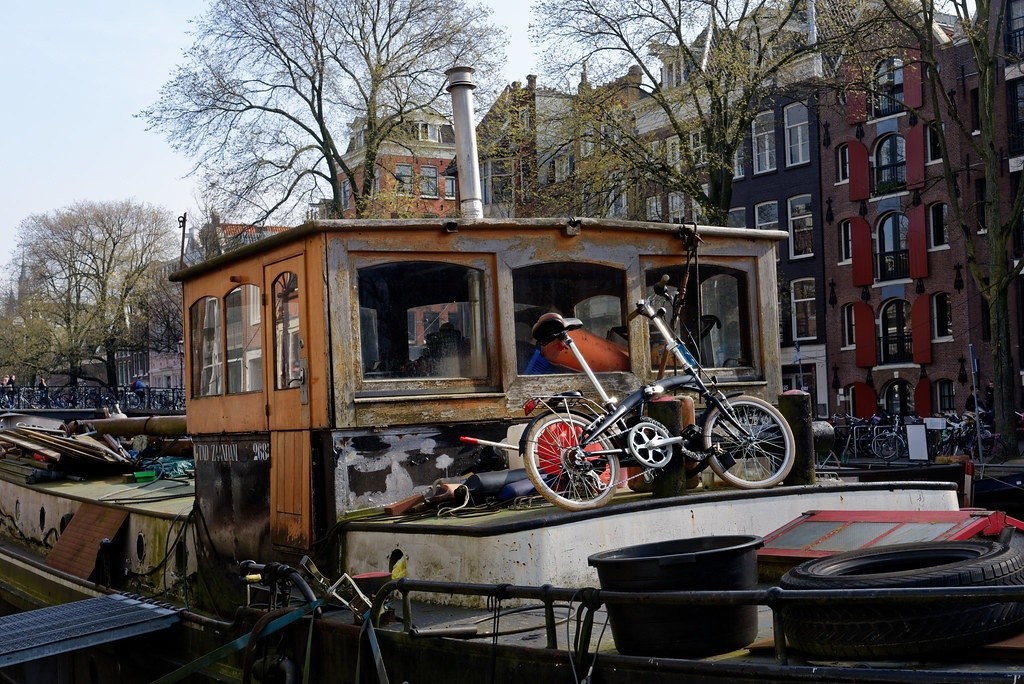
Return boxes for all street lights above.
[176,339,184,396]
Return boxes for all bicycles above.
[517,273,795,512]
[837,412,1009,472]
[0,382,185,412]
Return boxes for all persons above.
[965,381,994,413]
[129,374,145,404]
[38,379,47,409]
[0,375,15,409]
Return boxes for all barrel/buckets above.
[588,529,765,660]
